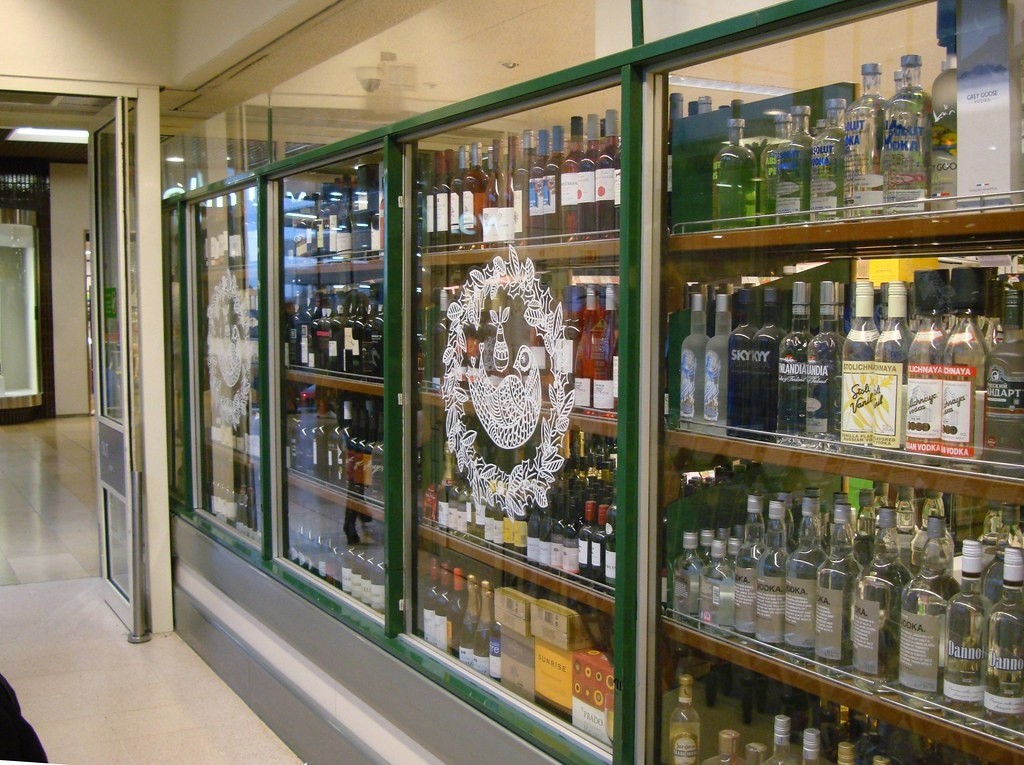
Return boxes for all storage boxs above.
[493,585,617,749]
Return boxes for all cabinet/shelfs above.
[168,0,1022,764]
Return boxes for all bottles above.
[195,55,1024,765]
